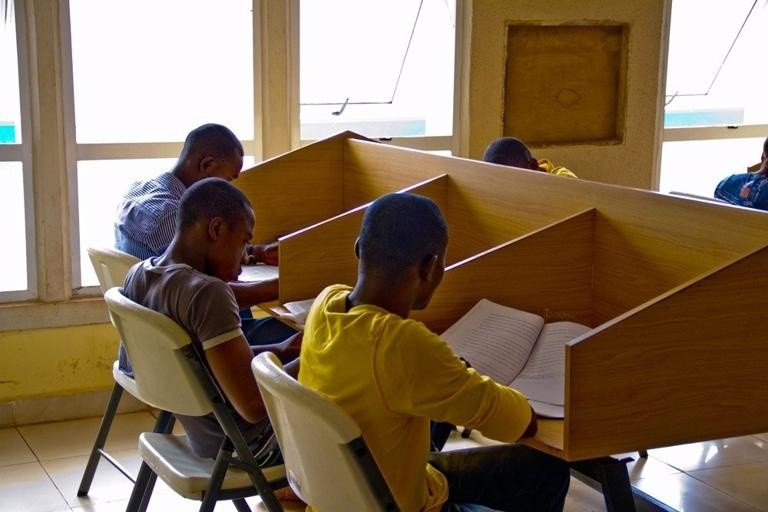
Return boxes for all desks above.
[229,129,768,512]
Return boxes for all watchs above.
[247,244,255,262]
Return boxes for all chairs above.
[251,351,400,512]
[77,246,177,512]
[103,285,284,512]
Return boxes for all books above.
[278,297,315,325]
[438,298,594,418]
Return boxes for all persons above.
[481,137,579,181]
[112,122,279,266]
[117,175,303,471]
[713,138,768,210]
[297,192,572,512]
